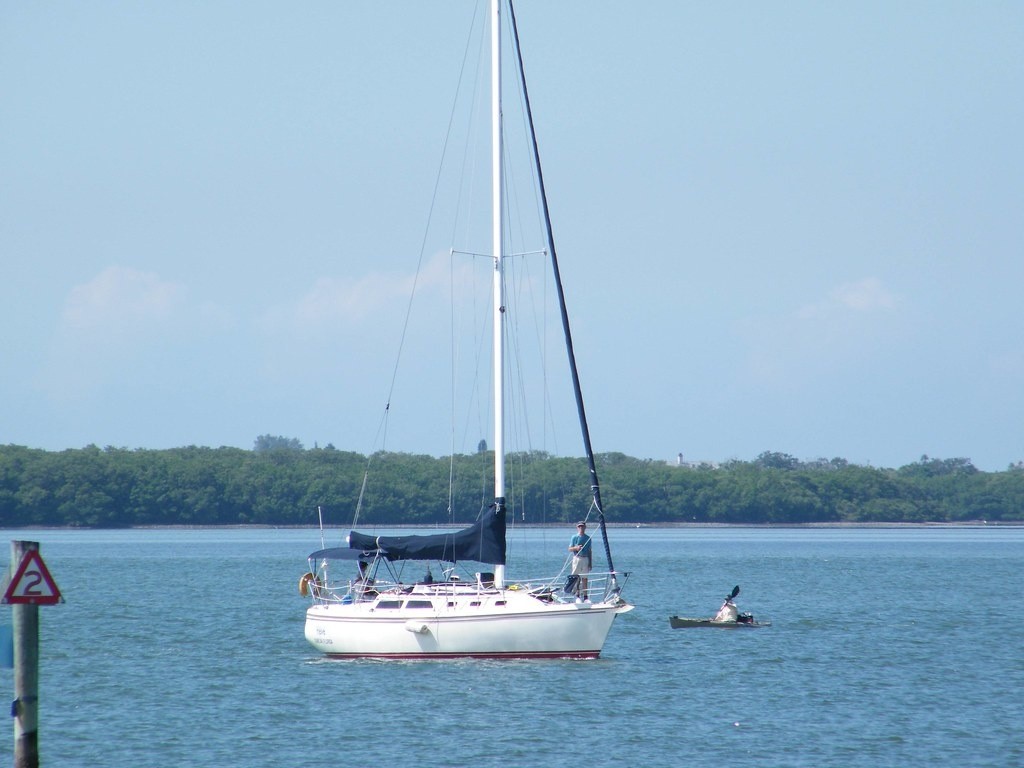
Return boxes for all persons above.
[569,521,593,604]
[354,561,374,596]
[717,595,737,623]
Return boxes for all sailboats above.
[300,1,635,658]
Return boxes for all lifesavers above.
[299,572,322,598]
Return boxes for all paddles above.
[712,585,740,621]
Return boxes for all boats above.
[670,615,771,628]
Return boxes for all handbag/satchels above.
[563,575,581,594]
[736,613,753,623]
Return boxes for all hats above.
[359,561,368,565]
[577,521,586,527]
